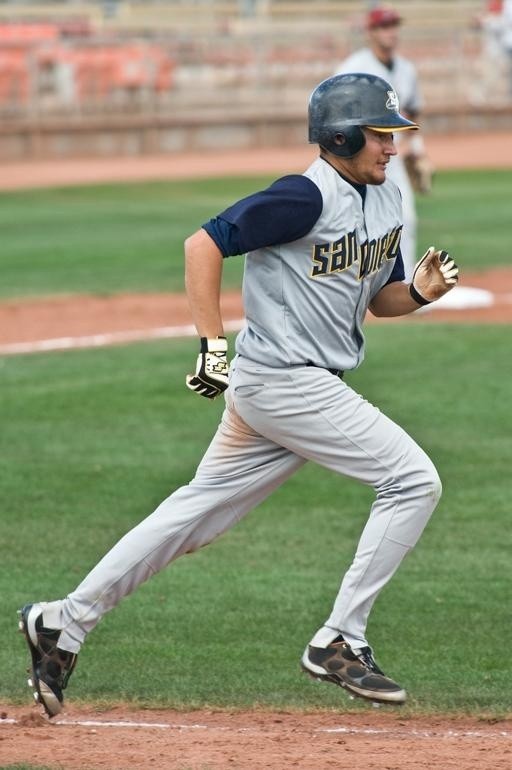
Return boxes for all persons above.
[26,46,85,114]
[16,70,459,723]
[332,6,435,291]
[468,0,512,57]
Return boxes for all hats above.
[366,6,401,31]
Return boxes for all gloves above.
[408,245,461,308]
[183,335,231,400]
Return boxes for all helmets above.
[305,70,422,160]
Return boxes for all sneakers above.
[15,594,79,720]
[298,624,408,706]
